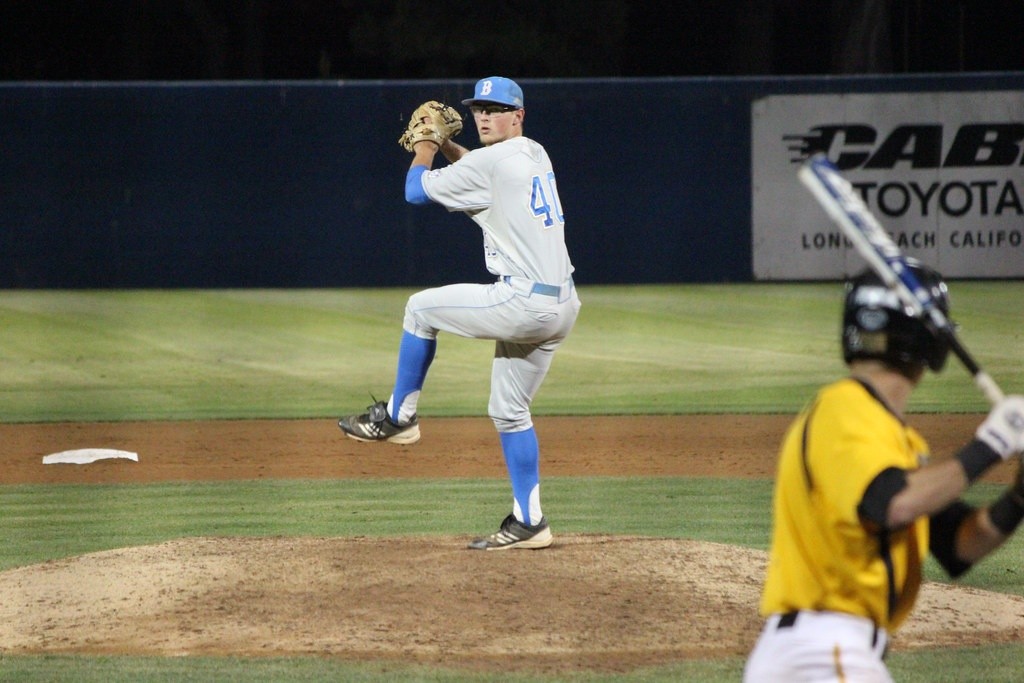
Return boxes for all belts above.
[498,276,574,297]
[776,611,888,660]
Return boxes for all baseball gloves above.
[398,99,463,153]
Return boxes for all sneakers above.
[338,391,420,444]
[467,512,553,551]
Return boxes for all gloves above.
[975,393,1024,460]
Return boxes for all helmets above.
[842,253,953,376]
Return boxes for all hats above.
[461,76,524,110]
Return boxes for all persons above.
[337,76,583,550]
[742,255,1024,683]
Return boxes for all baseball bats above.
[796,150,1006,413]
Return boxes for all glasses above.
[470,105,518,118]
